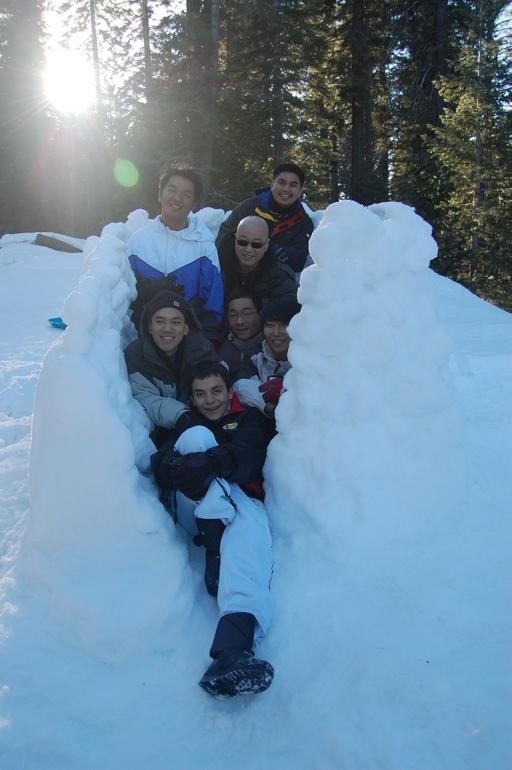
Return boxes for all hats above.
[146,294,188,321]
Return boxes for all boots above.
[200,647,274,697]
[192,519,220,595]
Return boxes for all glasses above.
[229,311,258,321]
[237,238,268,248]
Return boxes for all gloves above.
[162,443,225,503]
[260,376,284,404]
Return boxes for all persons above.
[220,214,300,326]
[128,289,232,529]
[157,358,278,699]
[214,162,317,271]
[212,291,265,385]
[124,160,225,349]
[228,296,299,419]
